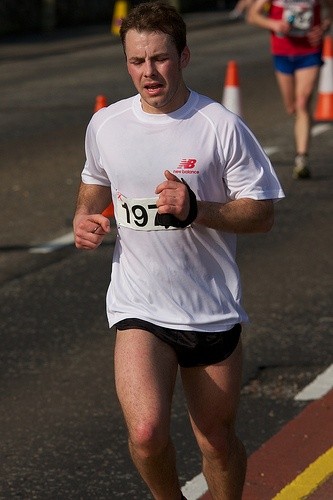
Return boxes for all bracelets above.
[155,177,198,228]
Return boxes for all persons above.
[246,0,333,179]
[73,0,285,500]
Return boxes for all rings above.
[93,226,100,233]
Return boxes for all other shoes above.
[292,155,310,179]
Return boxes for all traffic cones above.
[310,35,333,124]
[94,95,116,217]
[111,0,129,37]
[222,59,242,118]
